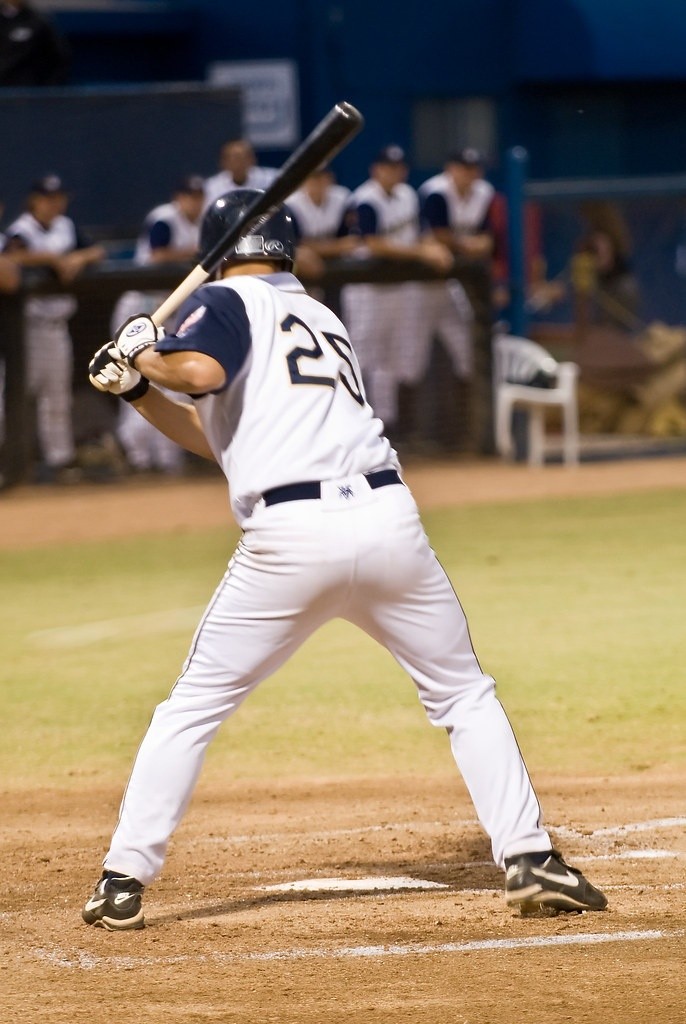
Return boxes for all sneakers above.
[82,870,145,931]
[506,848,608,911]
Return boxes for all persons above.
[0,136,634,484]
[81,189,607,929]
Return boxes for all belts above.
[263,469,403,506]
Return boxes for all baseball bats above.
[110,99,361,367]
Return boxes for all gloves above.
[114,312,167,371]
[88,340,150,402]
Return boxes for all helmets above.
[35,171,68,196]
[200,188,301,272]
[448,137,486,164]
[368,140,407,167]
[179,175,204,193]
